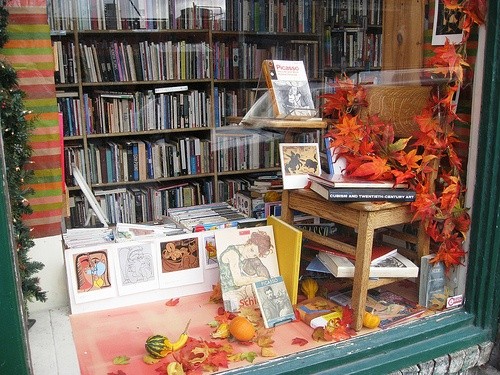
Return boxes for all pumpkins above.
[228,316,256,343]
[363,308,380,329]
[301,277,318,298]
[211,323,232,339]
[145,330,188,358]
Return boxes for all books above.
[326,287,428,329]
[419,253,444,308]
[293,296,342,330]
[253,275,296,328]
[303,233,419,278]
[47,0,417,249]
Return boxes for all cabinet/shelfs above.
[48,0,387,230]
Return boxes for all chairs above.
[279,84,443,333]
